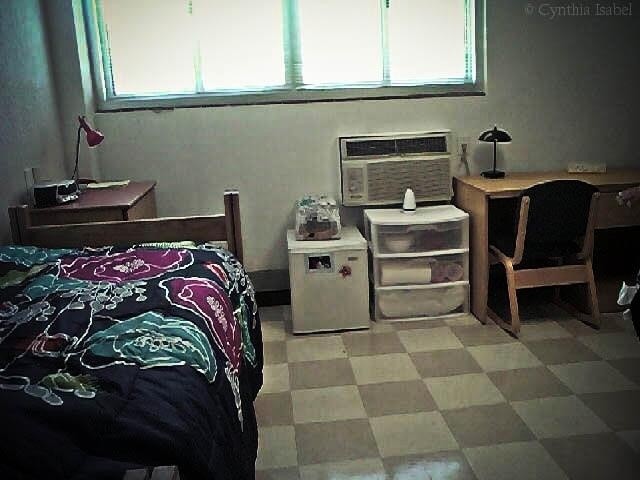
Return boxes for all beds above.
[0,187,265,478]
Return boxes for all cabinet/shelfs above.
[364,202,472,322]
[285,225,371,336]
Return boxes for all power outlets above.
[457,136,469,155]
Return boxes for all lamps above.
[477,125,514,179]
[67,114,106,179]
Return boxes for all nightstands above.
[24,179,160,227]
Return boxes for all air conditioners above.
[335,126,457,210]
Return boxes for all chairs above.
[487,177,601,338]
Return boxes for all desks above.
[453,166,639,329]
